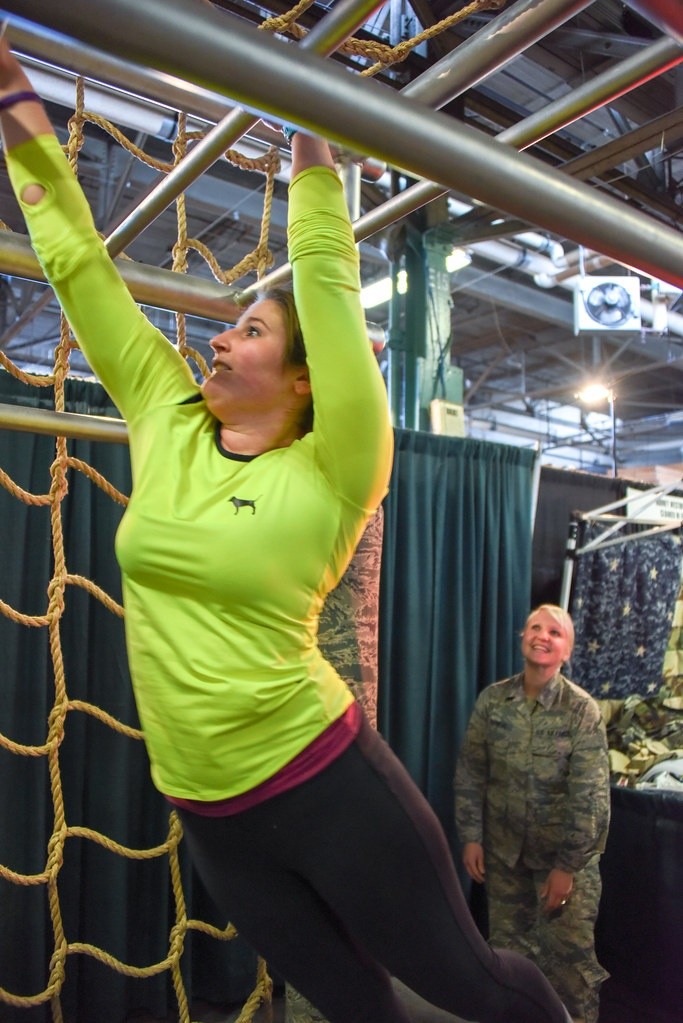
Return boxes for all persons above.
[0,36,572,1022]
[455,604,611,1023]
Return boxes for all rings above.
[561,900,566,905]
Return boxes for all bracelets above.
[282,126,297,146]
[0,92,43,109]
